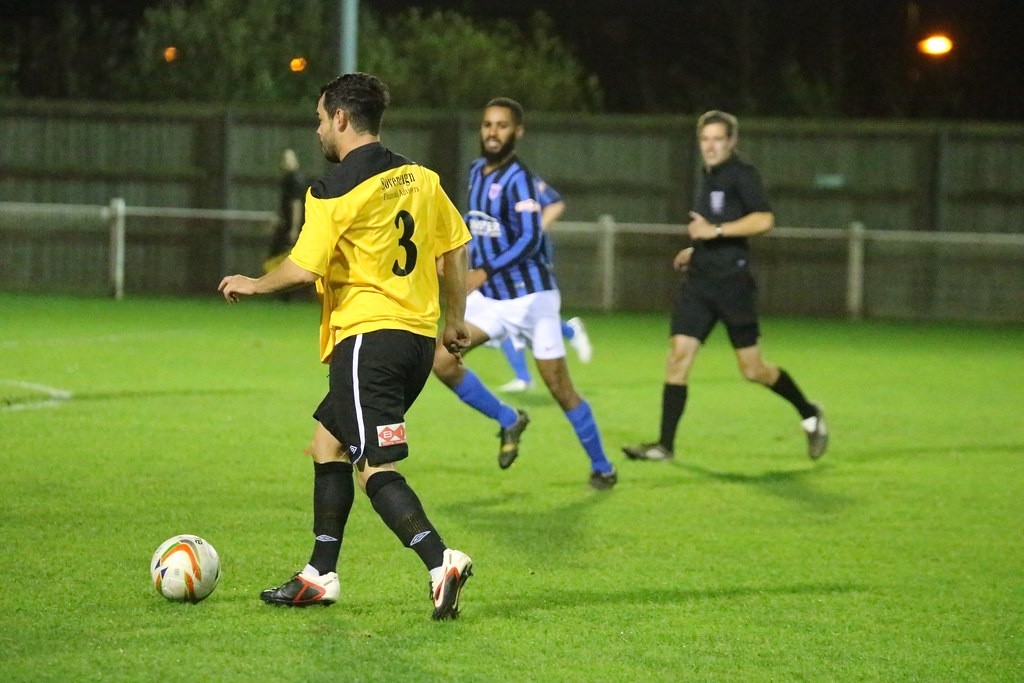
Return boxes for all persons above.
[218,73,474,621]
[431,99,618,490]
[265,149,305,307]
[622,109,829,462]
[499,175,593,393]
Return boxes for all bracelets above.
[716,223,721,237]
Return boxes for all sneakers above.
[590,469,617,490]
[429,548,473,622]
[623,440,673,461]
[801,404,827,458]
[260,563,340,608]
[496,410,530,469]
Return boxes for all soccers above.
[150,534,222,604]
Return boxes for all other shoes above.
[567,317,591,362]
[500,376,535,393]
